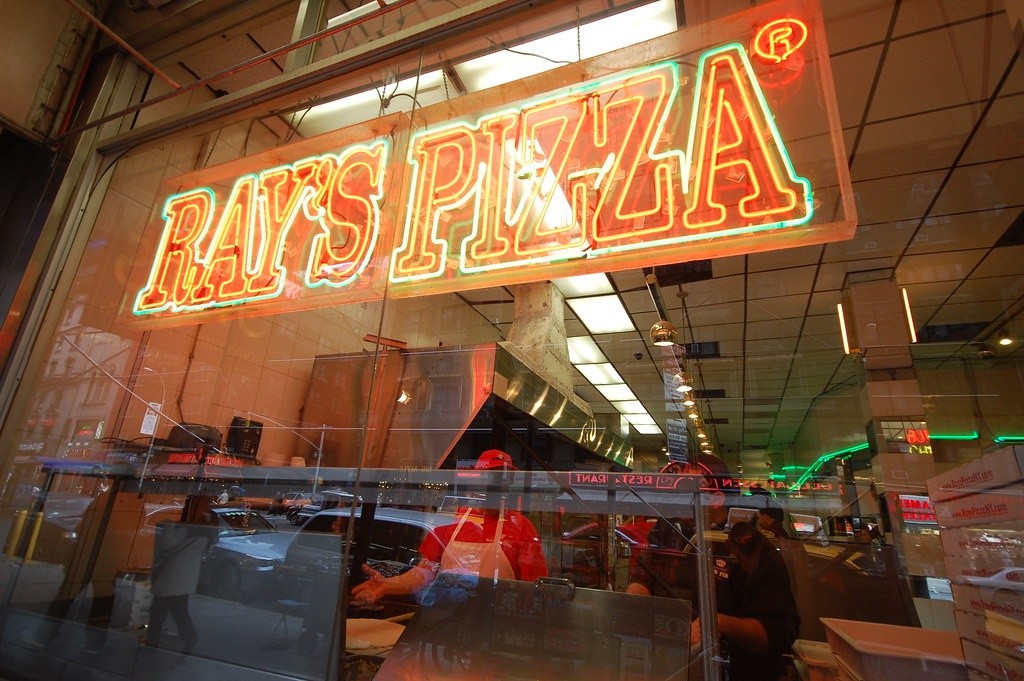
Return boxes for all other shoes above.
[184,634,199,653]
[20,630,47,652]
[139,640,157,650]
[75,638,103,656]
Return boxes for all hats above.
[475,449,519,470]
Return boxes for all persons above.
[21,461,146,654]
[840,525,885,602]
[217,490,229,505]
[267,491,285,515]
[748,484,785,535]
[352,449,550,605]
[134,491,220,656]
[624,455,800,681]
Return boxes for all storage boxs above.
[925,445,1024,681]
